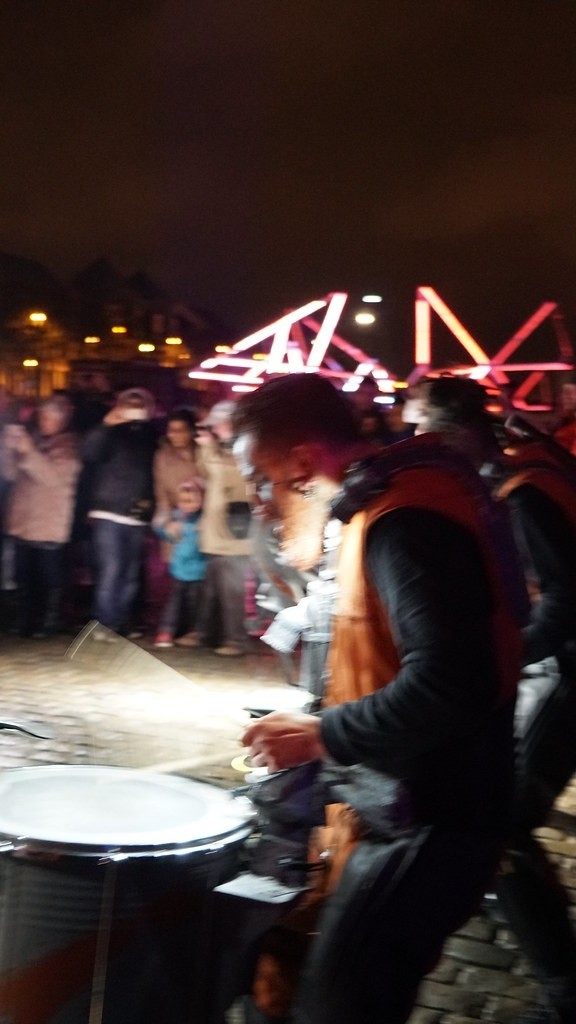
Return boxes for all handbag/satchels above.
[227,501,252,538]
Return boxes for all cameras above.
[192,426,212,438]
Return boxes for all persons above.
[0,363,576,1024]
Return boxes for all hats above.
[195,399,238,427]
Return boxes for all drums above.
[0,763,262,1024]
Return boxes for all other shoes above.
[32,628,48,639]
[118,630,144,639]
[153,633,173,649]
[213,642,246,657]
[174,631,208,648]
[9,624,26,635]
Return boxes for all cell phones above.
[4,425,25,437]
[122,407,146,420]
